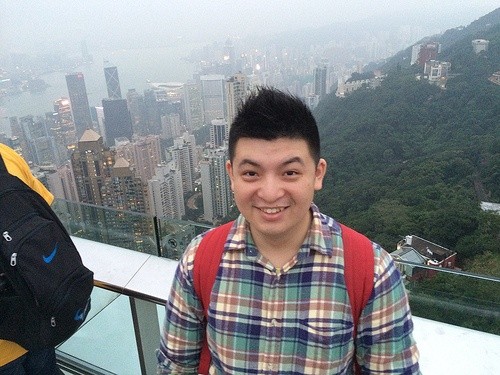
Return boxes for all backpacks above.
[0,171,94,351]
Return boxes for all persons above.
[0,142,67,375]
[155,86,423,375]
